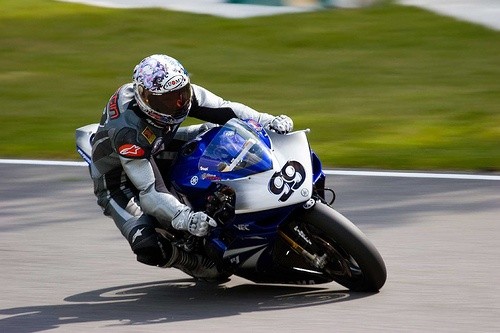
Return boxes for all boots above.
[161,244,220,279]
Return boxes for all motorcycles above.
[75,118,387,292]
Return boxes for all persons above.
[89,54,294,277]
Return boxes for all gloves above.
[269,114,294,133]
[171,207,218,238]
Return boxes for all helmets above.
[133,54,193,125]
